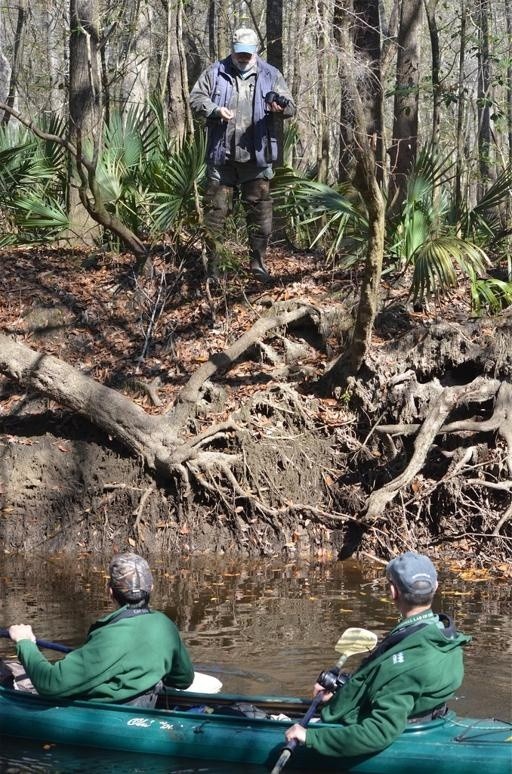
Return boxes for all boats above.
[0,662,512,774]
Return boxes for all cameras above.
[317,671,350,695]
[265,91,290,107]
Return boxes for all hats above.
[106,550,154,596]
[232,28,259,55]
[385,550,440,596]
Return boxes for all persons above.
[189,28,297,295]
[284,551,473,759]
[7,552,195,704]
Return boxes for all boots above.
[249,236,277,283]
[202,232,228,283]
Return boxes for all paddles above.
[0,628,224,695]
[270,626,377,772]
